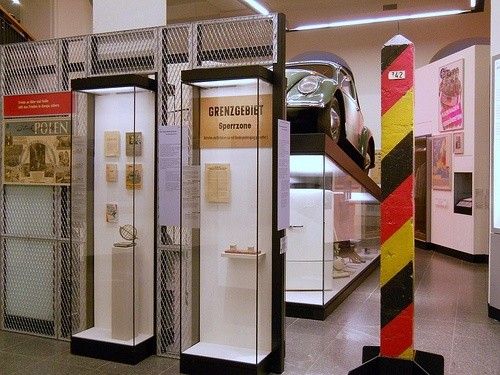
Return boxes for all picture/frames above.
[452,132,465,154]
[431,133,454,192]
[438,58,465,132]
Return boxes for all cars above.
[266,49,375,177]
